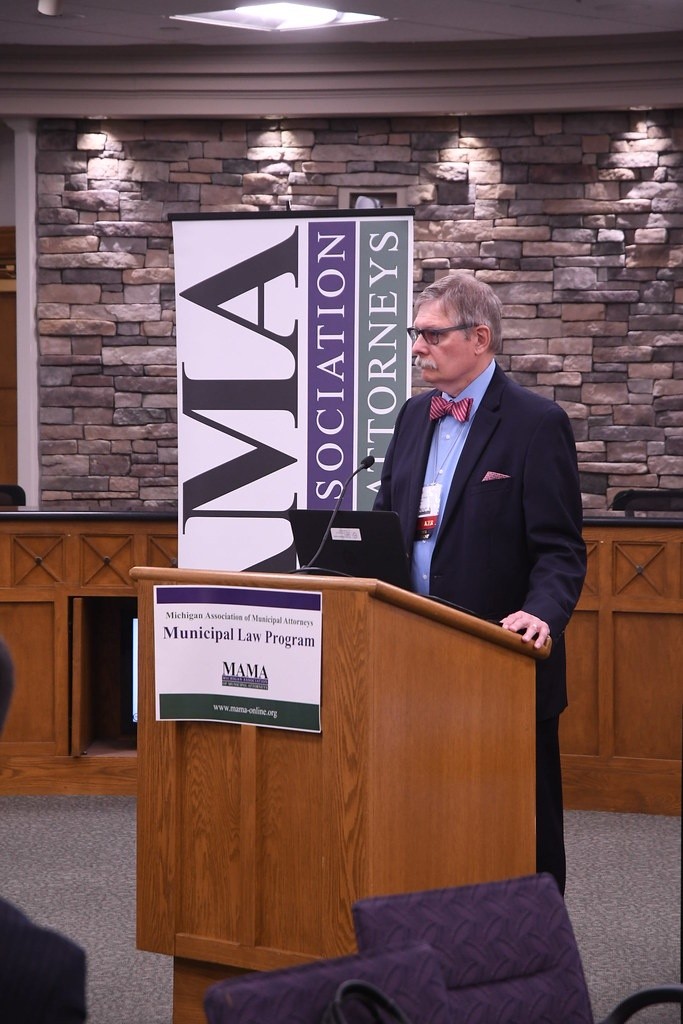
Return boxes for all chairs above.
[350,870,683,1024]
[0,484,26,506]
[612,489,683,511]
[201,942,454,1024]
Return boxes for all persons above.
[371,270,591,908]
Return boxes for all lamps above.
[36,0,64,17]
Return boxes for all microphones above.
[301,456,375,573]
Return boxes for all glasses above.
[407,323,479,345]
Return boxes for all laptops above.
[288,509,504,627]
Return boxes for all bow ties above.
[430,396,472,422]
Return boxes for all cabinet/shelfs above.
[0,511,683,817]
